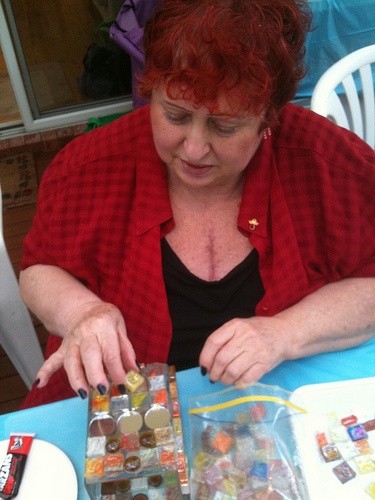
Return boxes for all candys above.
[190,403,291,500]
[314,414,375,492]
[85,370,187,500]
[0,432,33,500]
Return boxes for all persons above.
[17,0,375,413]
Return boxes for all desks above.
[0,336,375,500]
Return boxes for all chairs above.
[310,42,375,153]
